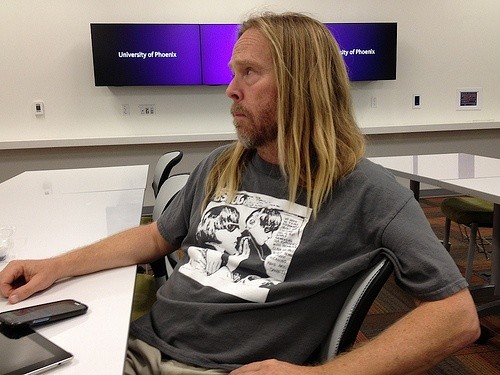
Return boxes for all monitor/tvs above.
[201,23,244,86]
[89,22,201,86]
[320,22,397,81]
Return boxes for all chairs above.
[441,196,495,282]
[130,151,393,375]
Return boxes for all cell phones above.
[0,298,87,329]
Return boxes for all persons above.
[0,12,482,375]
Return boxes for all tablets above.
[0,317,74,375]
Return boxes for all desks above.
[0,164,148,375]
[367,153,500,316]
